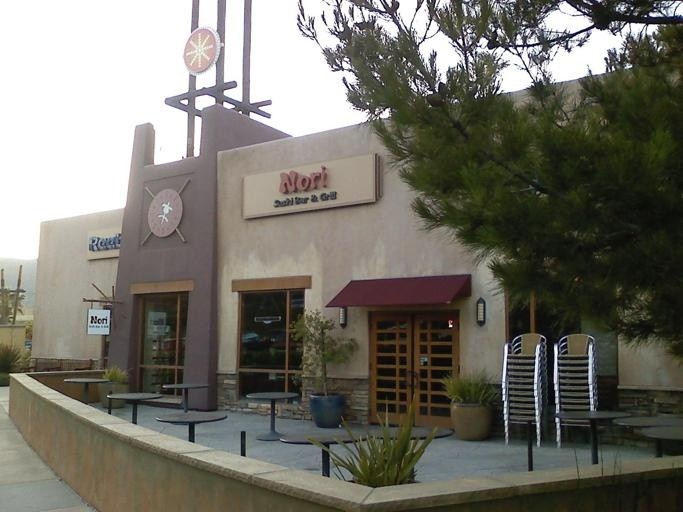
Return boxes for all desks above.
[64,377,110,405]
[162,384,209,413]
[553,411,632,464]
[106,392,164,424]
[639,426,683,440]
[154,412,228,442]
[246,391,299,441]
[611,416,683,457]
[279,431,367,477]
[366,426,454,440]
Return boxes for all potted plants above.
[442,367,501,440]
[287,306,359,428]
[1,341,23,386]
[100,367,129,408]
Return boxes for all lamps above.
[476,296,485,327]
[339,306,346,328]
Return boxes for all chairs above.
[501,333,600,447]
